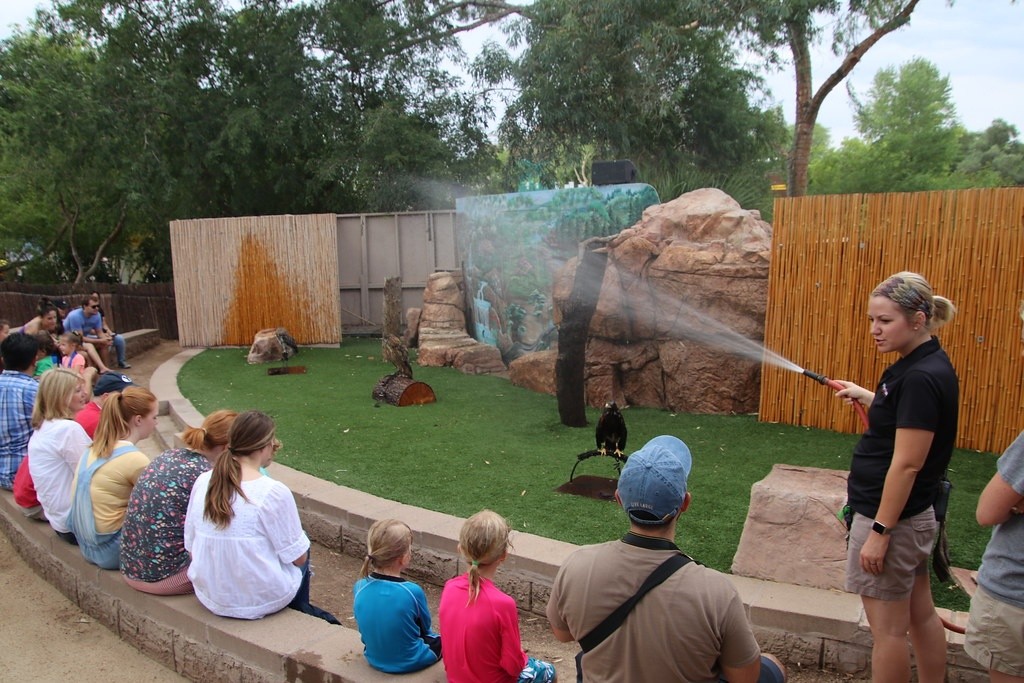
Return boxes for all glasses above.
[91,304,101,309]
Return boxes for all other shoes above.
[117,361,131,368]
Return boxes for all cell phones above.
[110,333,115,337]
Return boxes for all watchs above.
[871,521,892,536]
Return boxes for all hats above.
[617,435,693,525]
[93,370,140,395]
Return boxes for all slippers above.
[99,370,115,375]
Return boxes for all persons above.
[353,519,442,674]
[184,410,343,625]
[963,431,1024,683]
[828,270,961,683]
[545,434,786,683]
[119,410,237,596]
[0,290,138,545]
[438,511,558,683]
[65,386,159,571]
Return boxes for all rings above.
[870,562,877,565]
